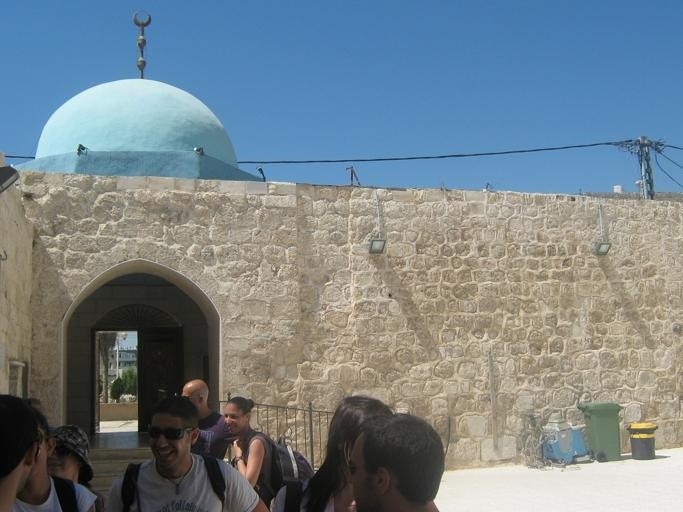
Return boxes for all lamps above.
[370,239,386,255]
[0,166,19,192]
[583,231,628,261]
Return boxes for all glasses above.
[147,426,193,439]
[53,448,75,456]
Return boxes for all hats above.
[50,425,94,482]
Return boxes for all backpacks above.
[243,432,314,489]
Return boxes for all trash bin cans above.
[577,402,624,461]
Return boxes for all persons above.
[270,398,393,512]
[103,396,269,512]
[28,397,56,432]
[345,410,444,512]
[220,397,280,507]
[14,412,98,512]
[49,424,109,512]
[0,394,42,512]
[180,379,228,459]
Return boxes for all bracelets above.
[231,455,243,464]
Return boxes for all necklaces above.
[159,458,193,496]
